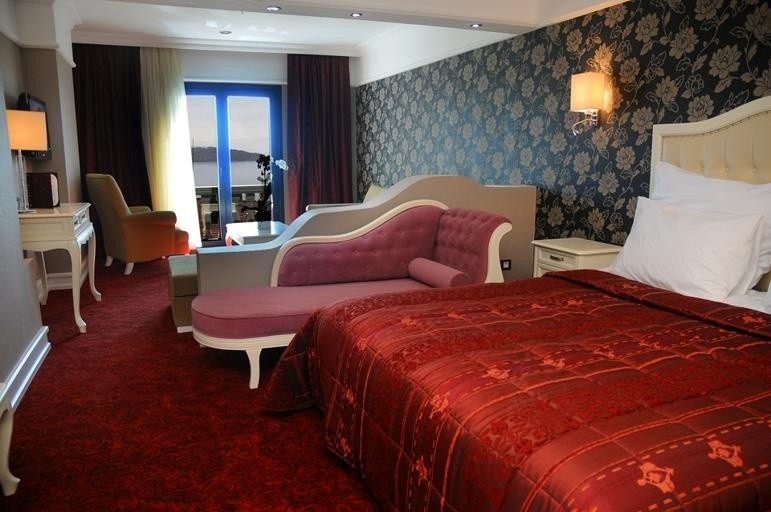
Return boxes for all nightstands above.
[527,234,621,278]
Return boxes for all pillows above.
[649,156,770,291]
[608,194,763,307]
[407,255,469,289]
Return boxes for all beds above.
[259,94,770,511]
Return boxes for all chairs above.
[305,183,386,213]
[84,170,189,275]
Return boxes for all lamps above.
[567,69,607,137]
[4,107,50,212]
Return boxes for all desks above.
[13,201,102,335]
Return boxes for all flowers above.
[239,153,291,222]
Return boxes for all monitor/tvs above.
[18,91,53,162]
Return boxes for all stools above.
[167,251,196,338]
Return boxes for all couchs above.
[187,197,516,389]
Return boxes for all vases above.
[257,220,271,230]
